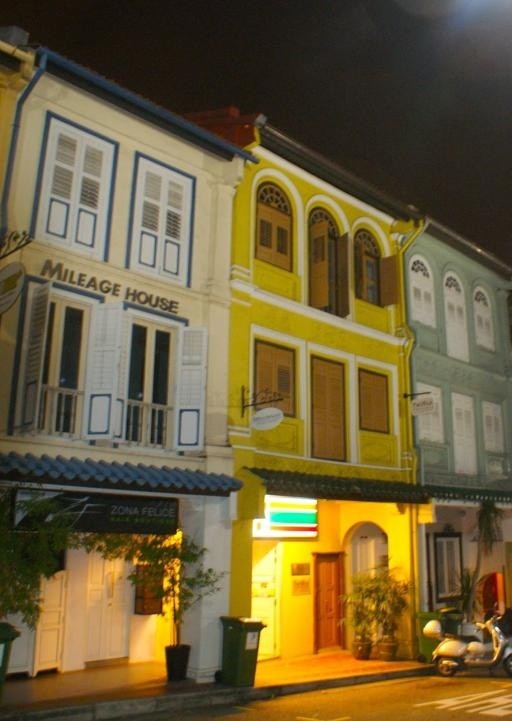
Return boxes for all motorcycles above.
[423,602,512,676]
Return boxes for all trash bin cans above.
[417,607,464,665]
[215,615,267,688]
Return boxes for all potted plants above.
[336,561,411,662]
[126,535,224,681]
